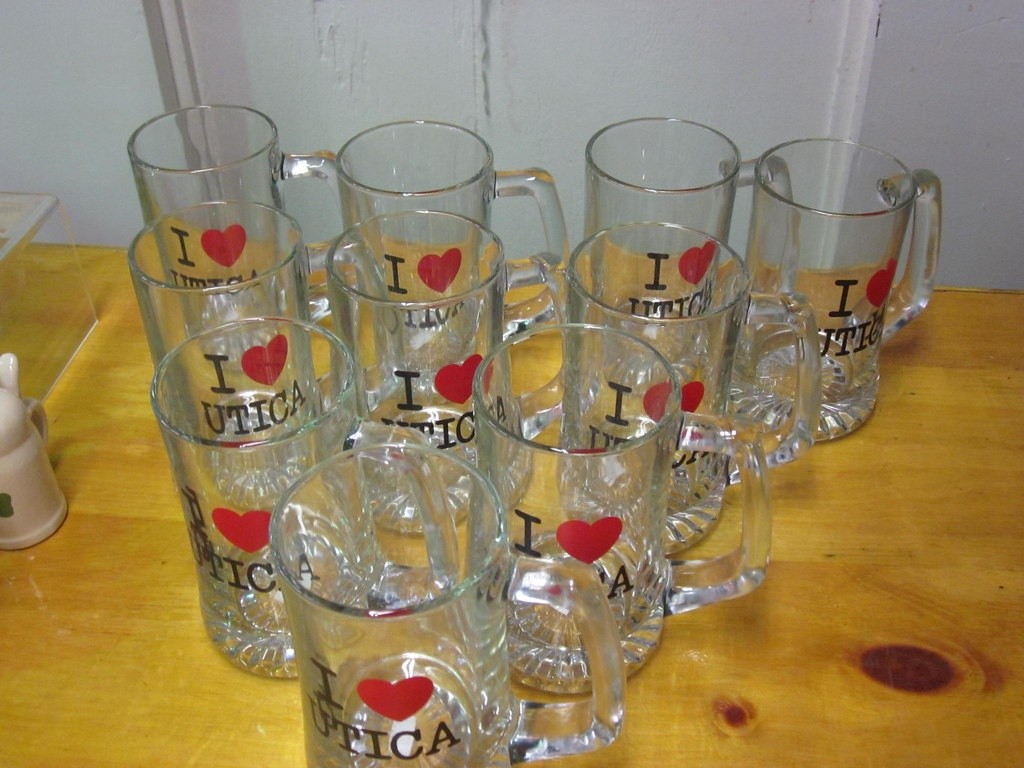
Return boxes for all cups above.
[125,94,943,768]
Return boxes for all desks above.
[0,243,1024,768]
